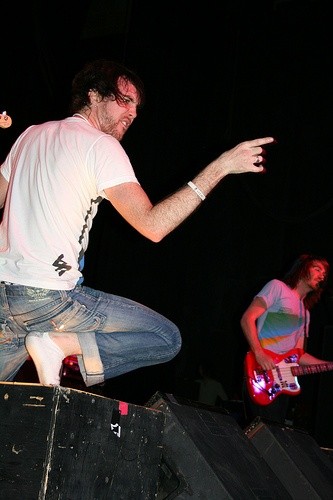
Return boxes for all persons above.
[0,58,274,386]
[240,254,333,426]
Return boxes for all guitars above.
[246,345,333,406]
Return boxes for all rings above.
[255,156,259,163]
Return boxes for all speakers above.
[0,380,333,500]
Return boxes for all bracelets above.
[188,181,206,201]
